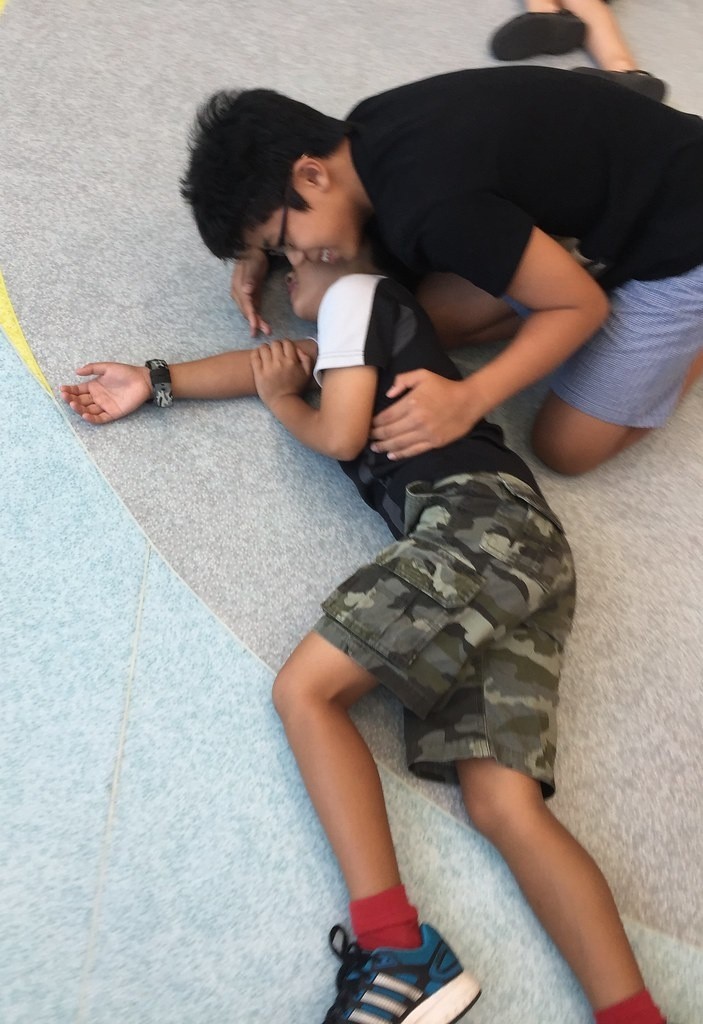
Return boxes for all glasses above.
[272,152,309,257]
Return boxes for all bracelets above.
[145,359,172,408]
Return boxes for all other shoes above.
[573,68,664,105]
[491,10,586,62]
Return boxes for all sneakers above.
[321,922,481,1024]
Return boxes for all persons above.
[489,1,664,102]
[176,65,703,478]
[60,257,665,1024]
